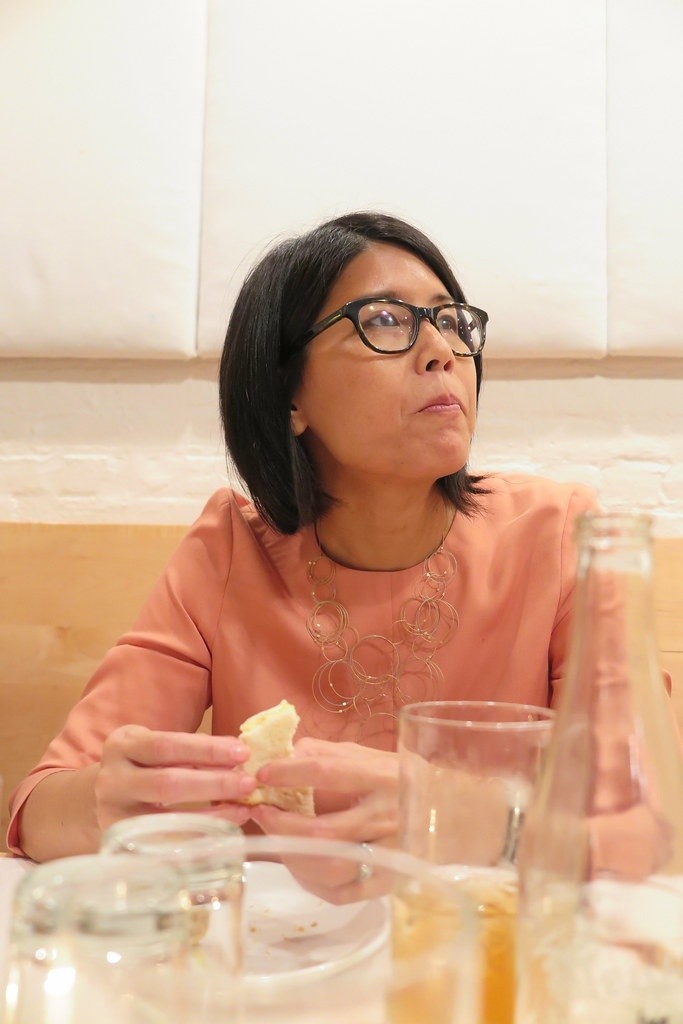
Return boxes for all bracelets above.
[507,804,523,864]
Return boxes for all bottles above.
[517,507,683,1024]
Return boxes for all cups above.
[398,697,551,1022]
[9,813,244,1023]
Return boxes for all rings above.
[360,843,375,881]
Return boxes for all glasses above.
[300,297,489,357]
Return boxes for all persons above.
[5,211,676,906]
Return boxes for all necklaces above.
[302,508,461,744]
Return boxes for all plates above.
[124,858,392,996]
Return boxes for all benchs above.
[0,519,683,885]
